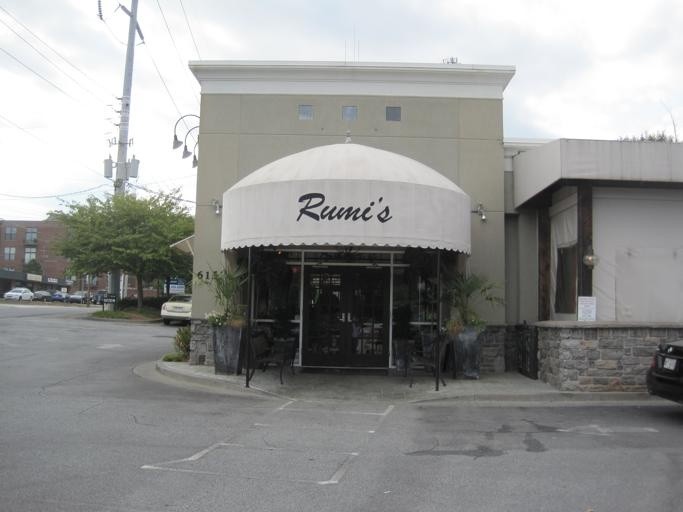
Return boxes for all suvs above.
[70,290,94,304]
[90,289,108,305]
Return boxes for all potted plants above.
[185,251,248,376]
[428,262,507,379]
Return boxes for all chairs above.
[401,336,446,387]
[243,326,289,384]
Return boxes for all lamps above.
[583,245,599,270]
[173,114,200,168]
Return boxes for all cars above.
[32,290,53,302]
[3,287,34,302]
[52,291,71,303]
[359,309,424,342]
[160,293,193,326]
[646,339,683,405]
